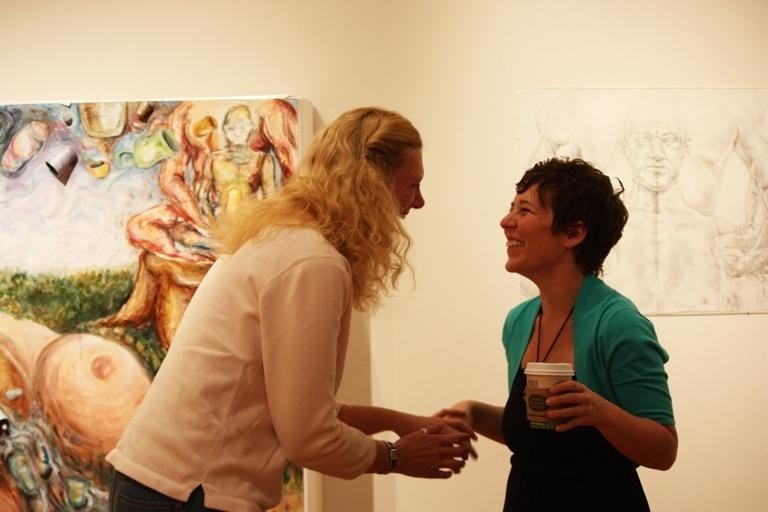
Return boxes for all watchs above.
[384,435,398,476]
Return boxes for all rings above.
[421,426,427,433]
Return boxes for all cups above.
[524,361,575,430]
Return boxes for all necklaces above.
[536,304,576,363]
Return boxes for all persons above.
[105,107,467,511]
[90,99,295,325]
[607,113,732,311]
[428,154,678,512]
